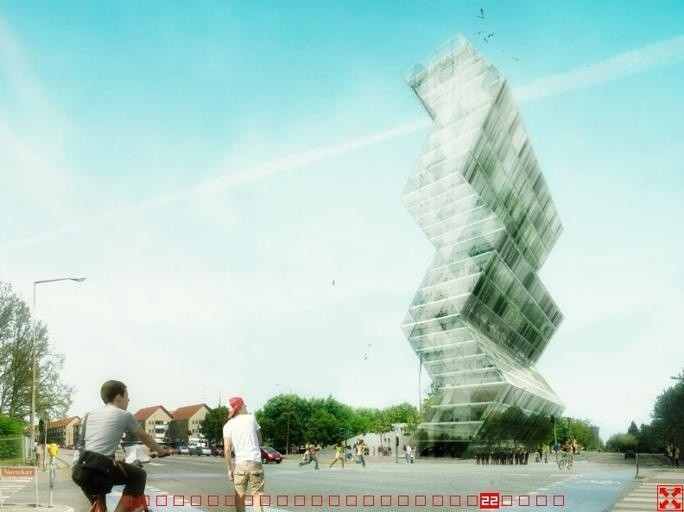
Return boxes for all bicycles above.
[90,453,167,512]
[558,451,574,470]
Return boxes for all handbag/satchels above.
[77,451,117,494]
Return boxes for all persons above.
[34,444,43,467]
[298,438,416,472]
[222,397,265,512]
[667,442,680,469]
[475,439,575,465]
[48,443,59,465]
[72,380,170,512]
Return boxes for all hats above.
[228,397,243,419]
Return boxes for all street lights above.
[33,277,84,507]
[567,417,572,435]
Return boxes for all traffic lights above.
[38,419,47,433]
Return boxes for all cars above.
[260,447,283,463]
[162,445,234,458]
